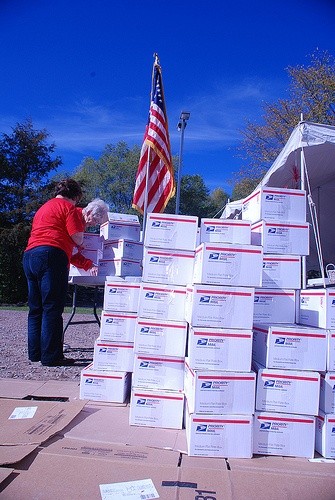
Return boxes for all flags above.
[132,57,176,216]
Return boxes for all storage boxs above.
[68,187,335,460]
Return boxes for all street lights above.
[175,112,191,215]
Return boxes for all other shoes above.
[43,358,74,366]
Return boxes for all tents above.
[220,112,335,288]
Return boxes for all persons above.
[62,198,109,352]
[22,178,84,367]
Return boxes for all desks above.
[62,276,106,344]
